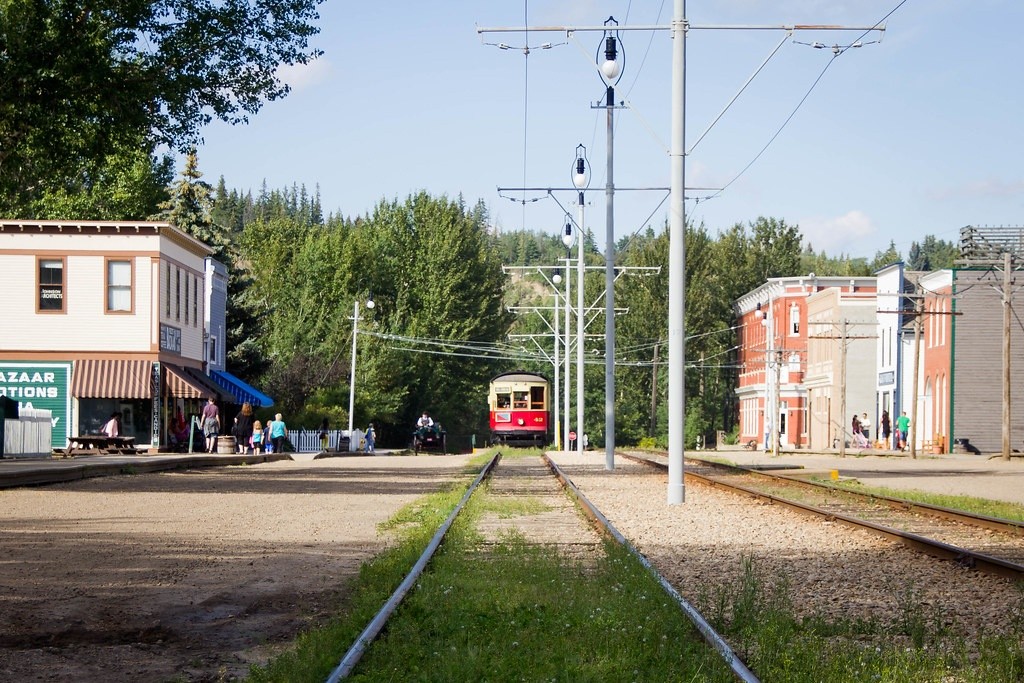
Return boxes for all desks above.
[63,436,135,459]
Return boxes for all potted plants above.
[933,433,943,454]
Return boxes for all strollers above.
[853,430,872,448]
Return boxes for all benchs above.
[52,447,137,458]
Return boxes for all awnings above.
[73,360,153,401]
[161,362,273,406]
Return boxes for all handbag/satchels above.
[232,427,237,434]
[319,432,325,439]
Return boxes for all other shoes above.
[209,451,213,454]
[205,448,208,452]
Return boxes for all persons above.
[234,402,288,455]
[364,423,376,453]
[417,412,434,428]
[103,411,122,438]
[317,418,330,452]
[852,410,910,453]
[200,395,220,452]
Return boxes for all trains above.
[487,369,552,448]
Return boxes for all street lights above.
[561,211,576,453]
[571,142,593,452]
[591,14,625,471]
[349,299,375,438]
[551,263,563,449]
